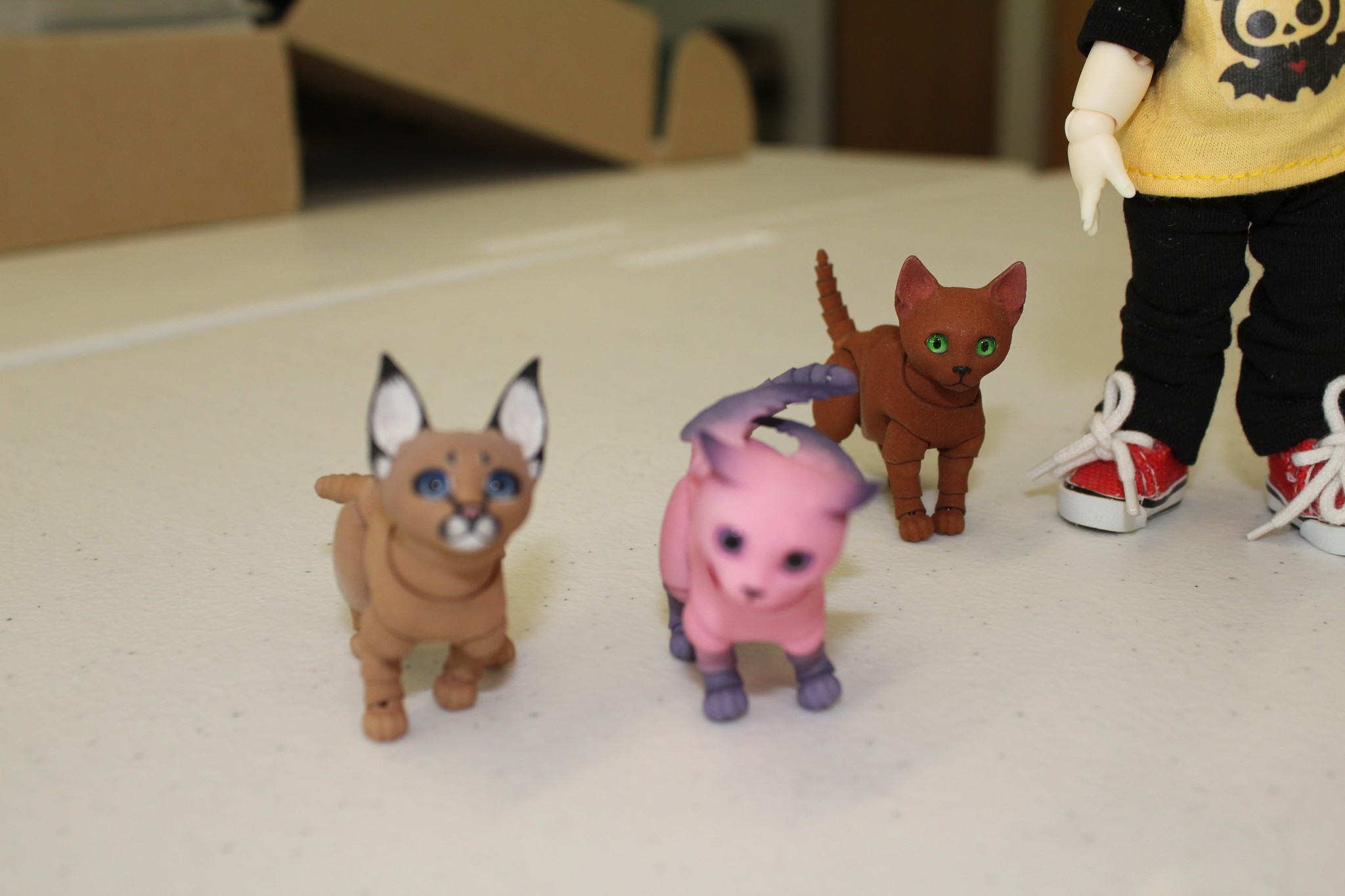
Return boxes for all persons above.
[1015,0,1345,557]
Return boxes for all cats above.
[657,359,871,724]
[812,249,1026,542]
[314,354,551,744]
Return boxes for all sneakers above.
[1027,368,1189,531]
[1246,374,1345,557]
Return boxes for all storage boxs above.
[0,0,751,254]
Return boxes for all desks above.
[0,147,1345,896]
